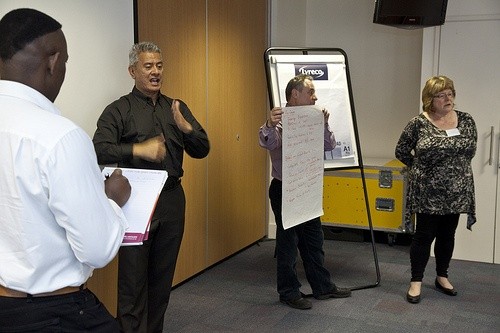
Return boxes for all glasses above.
[433,92,453,99]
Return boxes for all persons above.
[92,43,210,333]
[0,8,131,333]
[395,76,478,303]
[259,75,351,310]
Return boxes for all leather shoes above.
[280,289,312,310]
[435,277,458,295]
[407,283,421,302]
[312,285,352,300]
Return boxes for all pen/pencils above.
[105,173,109,179]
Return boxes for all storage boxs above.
[319,158,415,246]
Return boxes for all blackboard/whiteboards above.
[264,45,363,171]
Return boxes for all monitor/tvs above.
[373,0,448,26]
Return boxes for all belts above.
[0,282,88,297]
